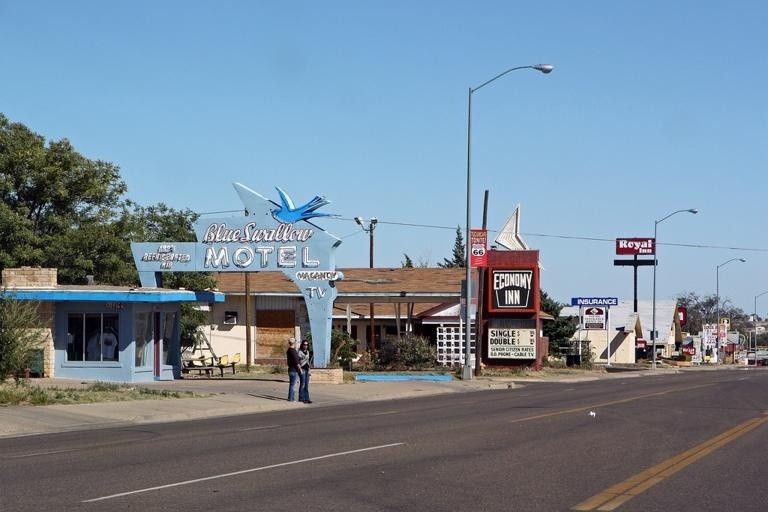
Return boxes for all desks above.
[182,357,212,378]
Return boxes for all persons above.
[297,340,313,404]
[287,337,298,402]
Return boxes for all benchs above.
[185,367,214,376]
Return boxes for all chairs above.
[211,355,229,377]
[226,353,241,374]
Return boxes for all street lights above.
[354,216,378,354]
[651,208,698,370]
[462,63,554,380]
[717,257,746,367]
